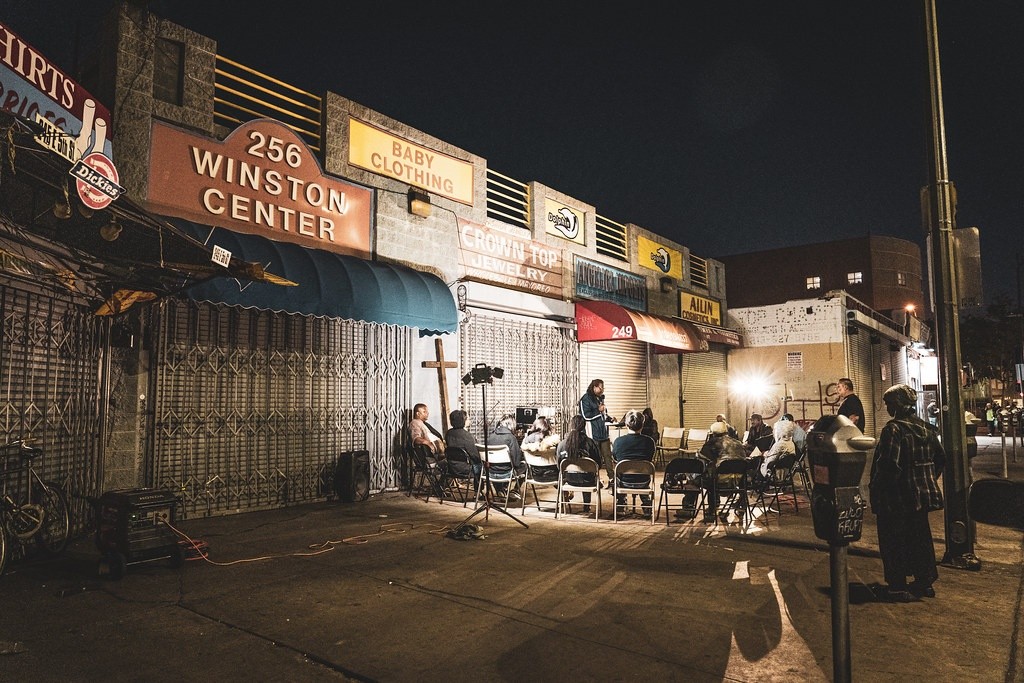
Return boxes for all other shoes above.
[616,507,626,515]
[878,589,910,601]
[473,492,484,502]
[582,511,590,518]
[906,583,935,597]
[433,487,451,496]
[509,489,521,500]
[705,508,717,519]
[498,488,507,503]
[643,507,651,516]
[676,509,694,516]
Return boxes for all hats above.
[709,422,726,435]
[625,408,645,426]
[882,384,916,408]
[449,410,467,426]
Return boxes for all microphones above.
[601,395,605,404]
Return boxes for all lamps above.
[407,187,432,218]
[660,276,674,292]
[54,189,122,241]
[455,361,531,529]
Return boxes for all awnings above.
[575,300,710,355]
[145,212,458,338]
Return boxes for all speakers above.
[336,450,369,500]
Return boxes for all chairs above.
[408,427,810,528]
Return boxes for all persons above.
[642,407,660,443]
[836,377,866,434]
[577,379,618,489]
[484,414,527,500]
[444,409,486,501]
[675,414,807,521]
[520,415,575,500]
[981,402,998,437]
[867,382,947,603]
[406,402,454,497]
[926,398,940,428]
[611,409,656,520]
[556,414,605,514]
